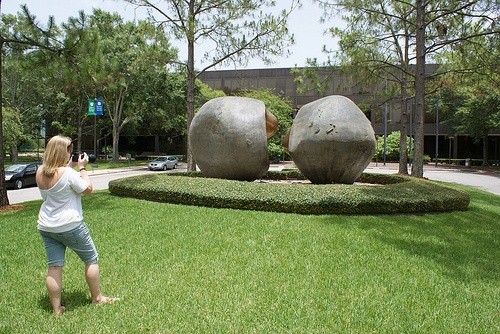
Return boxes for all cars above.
[3,163,42,190]
[148,155,178,170]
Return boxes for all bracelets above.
[79,167,86,171]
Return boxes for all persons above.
[35,135,118,319]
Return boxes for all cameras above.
[71,154,96,163]
